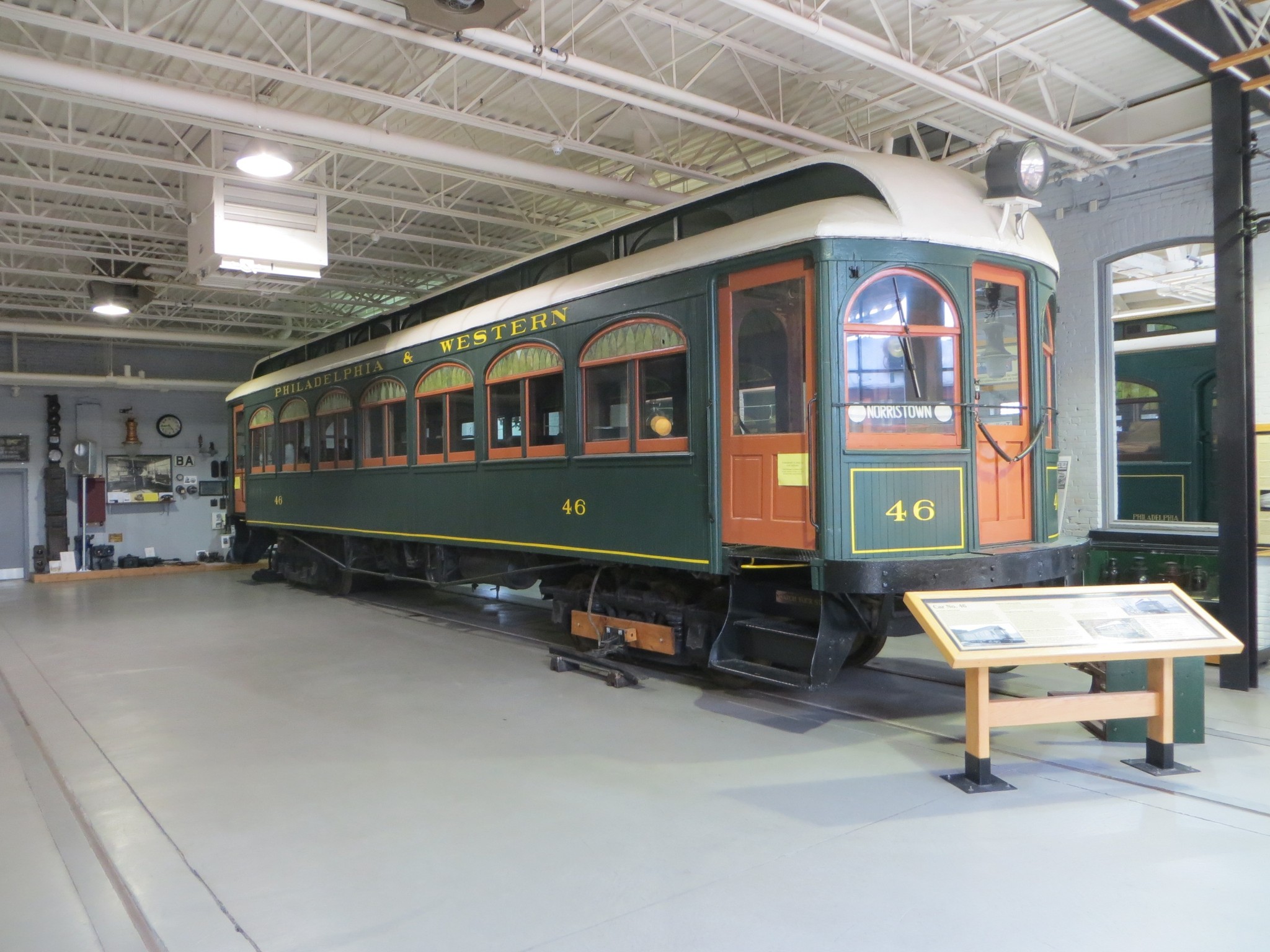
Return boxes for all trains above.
[1092,302,1220,603]
[223,138,1090,688]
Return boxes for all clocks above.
[156,414,182,437]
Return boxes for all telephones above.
[161,495,174,501]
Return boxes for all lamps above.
[236,138,293,177]
[989,138,1049,200]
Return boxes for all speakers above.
[46,515,69,562]
[92,545,115,559]
[33,545,46,573]
[43,468,67,516]
